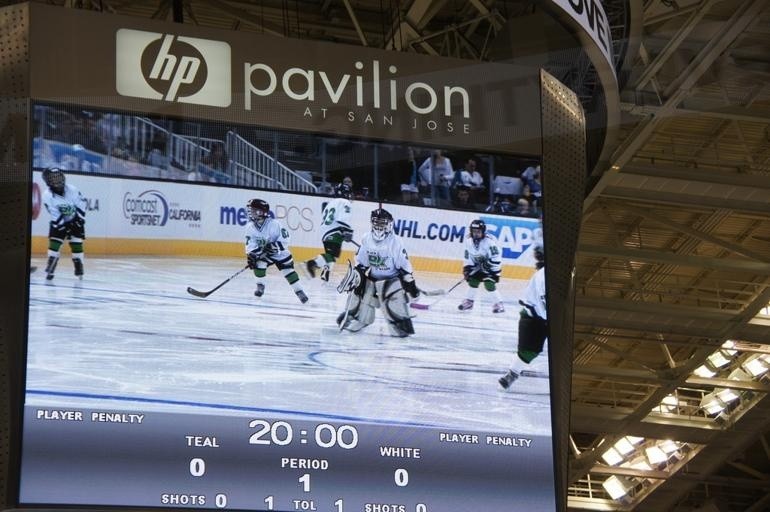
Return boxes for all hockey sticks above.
[409,270,476,310]
[418,288,445,296]
[187,266,249,298]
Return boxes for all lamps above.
[602,341,768,501]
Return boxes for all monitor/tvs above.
[13,95,559,512]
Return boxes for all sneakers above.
[295,289,309,304]
[46,271,54,280]
[498,369,519,389]
[321,264,330,283]
[458,298,474,311]
[491,301,504,312]
[254,283,266,297]
[74,264,83,275]
[306,259,318,278]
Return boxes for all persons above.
[304,183,354,281]
[498,245,548,389]
[336,209,419,337]
[245,200,308,304]
[459,219,506,312]
[41,167,90,286]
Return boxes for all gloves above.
[350,267,367,296]
[261,243,278,258]
[399,274,420,306]
[246,251,260,272]
[479,261,494,276]
[463,265,474,281]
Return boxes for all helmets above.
[470,220,486,240]
[40,166,67,195]
[247,198,270,220]
[369,207,395,238]
[334,182,352,200]
